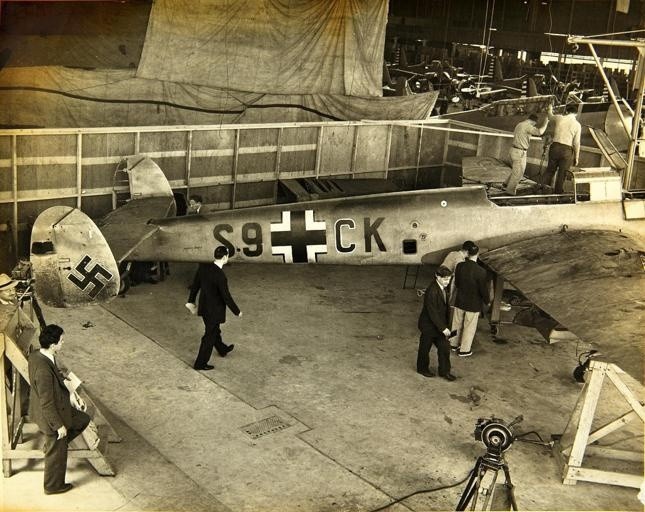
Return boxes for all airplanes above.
[22,148,645,395]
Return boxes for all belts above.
[552,141,574,150]
[511,143,528,152]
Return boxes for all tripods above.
[456,461,520,511]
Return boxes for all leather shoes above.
[43,483,73,495]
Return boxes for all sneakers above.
[417,367,436,377]
[450,343,461,351]
[194,364,214,370]
[439,372,456,381]
[457,351,473,357]
[220,344,234,357]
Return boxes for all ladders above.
[1,305,120,477]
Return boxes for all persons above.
[450,245,490,357]
[188,194,209,215]
[502,113,550,196]
[28,324,90,494]
[418,265,457,381]
[547,103,581,194]
[188,246,243,370]
[441,241,474,340]
